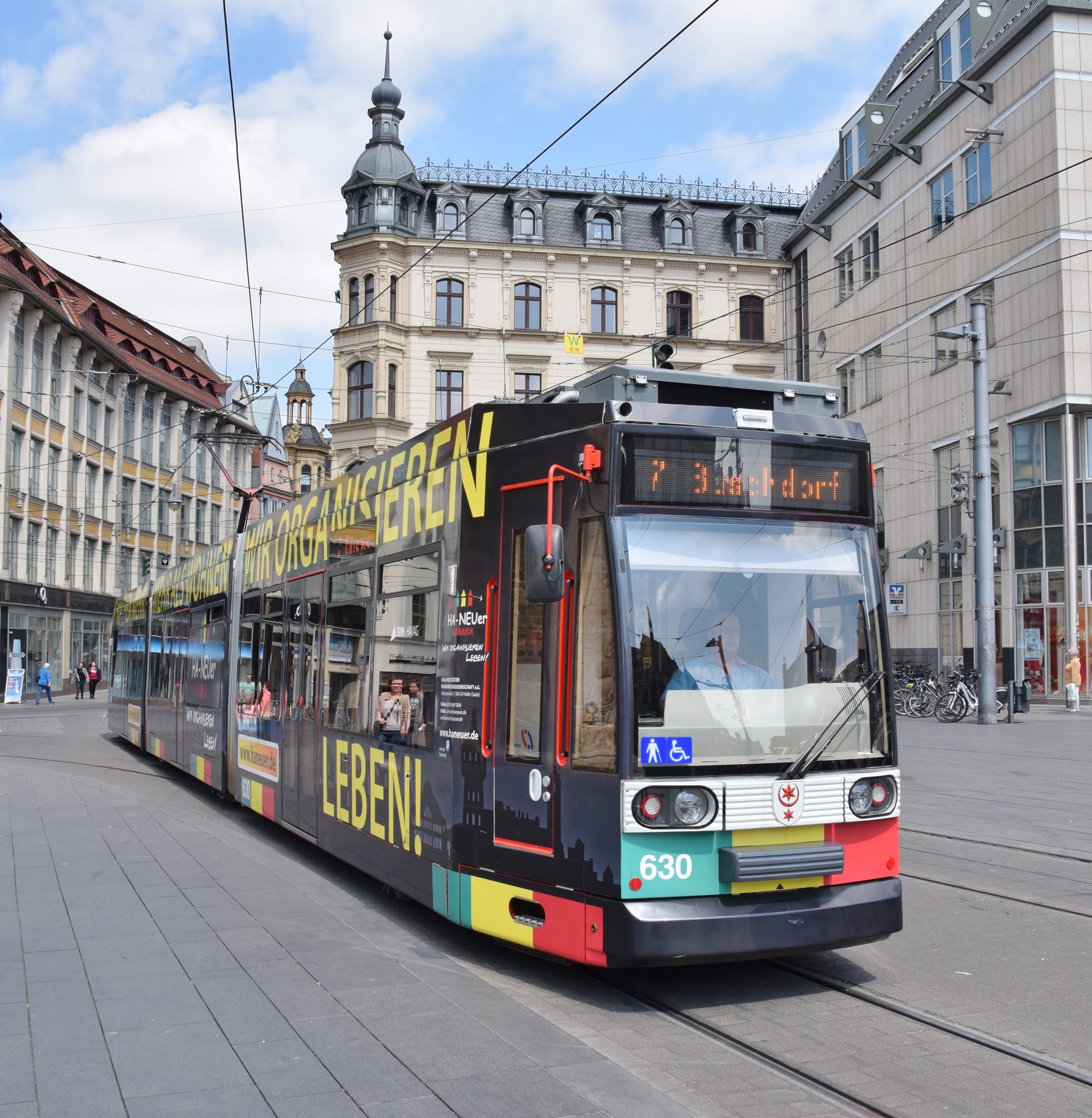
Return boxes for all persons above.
[236,673,271,718]
[70,662,89,699]
[1028,628,1044,692]
[35,663,55,705]
[87,661,101,699]
[1064,647,1082,712]
[377,676,426,747]
[659,610,783,711]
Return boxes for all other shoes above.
[1070,709,1080,712]
[75,696,78,700]
[49,702,55,704]
[81,697,84,699]
[1067,708,1073,711]
[90,696,92,699]
[92,696,95,699]
[36,703,40,705]
[1031,688,1034,692]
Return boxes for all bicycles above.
[885,659,1011,723]
[814,661,867,686]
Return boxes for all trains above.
[108,368,904,973]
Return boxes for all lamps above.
[184,523,189,553]
[988,381,1012,395]
[165,482,184,511]
[951,467,968,485]
[964,129,1004,148]
[125,525,131,542]
[76,508,82,527]
[951,481,968,493]
[951,494,965,505]
[16,491,23,509]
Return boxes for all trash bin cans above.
[1014,678,1032,714]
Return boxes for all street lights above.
[930,302,1001,726]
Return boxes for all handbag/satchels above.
[35,676,39,683]
[245,695,251,709]
[1065,683,1076,702]
[373,720,382,737]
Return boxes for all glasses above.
[263,687,267,689]
